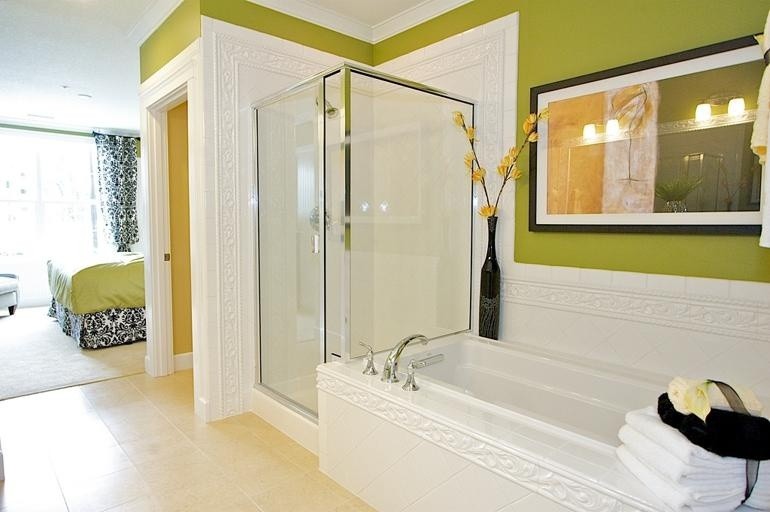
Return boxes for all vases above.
[478,215,502,341]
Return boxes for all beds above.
[45,250,147,348]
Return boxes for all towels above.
[619,376,769,512]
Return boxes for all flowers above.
[453,106,553,215]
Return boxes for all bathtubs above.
[359,332,770,457]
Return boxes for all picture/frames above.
[527,33,770,236]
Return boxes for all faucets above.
[381,333,429,384]
